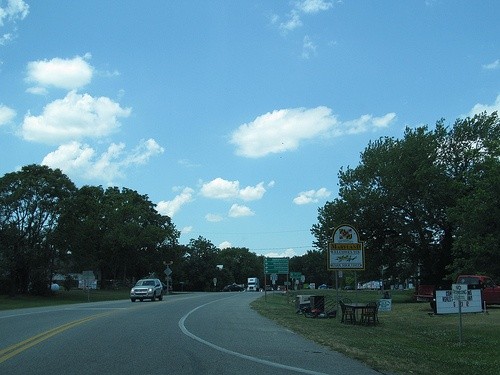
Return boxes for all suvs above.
[456,275,500,308]
[129,279,164,301]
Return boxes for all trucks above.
[247,278,260,292]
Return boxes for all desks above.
[344,303,373,322]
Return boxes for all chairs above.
[338,298,380,326]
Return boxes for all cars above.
[318,284,329,289]
[223,286,244,292]
